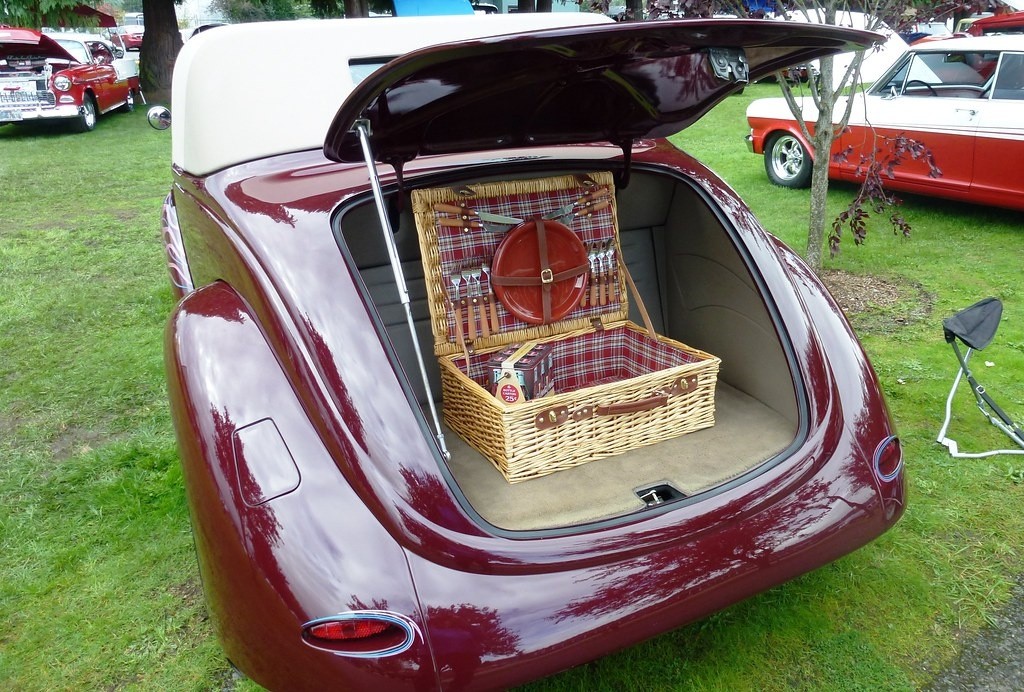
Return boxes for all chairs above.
[93,49,112,65]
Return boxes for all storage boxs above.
[485,342,555,405]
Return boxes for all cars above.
[0,26,140,131]
[905,10,997,37]
[101,25,147,52]
[745,8,1024,210]
[145,13,908,692]
